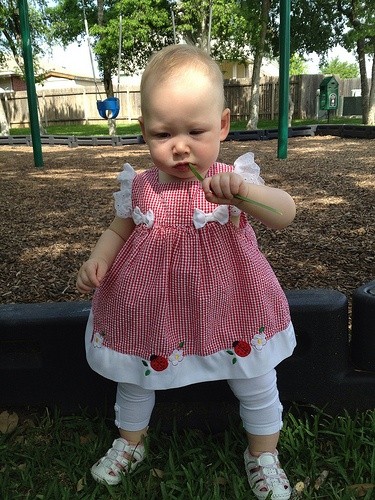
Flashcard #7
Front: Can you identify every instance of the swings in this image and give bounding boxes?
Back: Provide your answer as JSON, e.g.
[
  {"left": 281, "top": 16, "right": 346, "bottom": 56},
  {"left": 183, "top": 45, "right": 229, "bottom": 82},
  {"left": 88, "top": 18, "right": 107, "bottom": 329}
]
[
  {"left": 82, "top": 0, "right": 124, "bottom": 121},
  {"left": 169, "top": 0, "right": 214, "bottom": 59}
]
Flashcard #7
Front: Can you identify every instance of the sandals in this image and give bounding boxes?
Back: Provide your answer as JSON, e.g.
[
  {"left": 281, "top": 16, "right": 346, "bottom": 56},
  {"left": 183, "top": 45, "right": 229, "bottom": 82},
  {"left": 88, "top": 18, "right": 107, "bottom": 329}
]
[
  {"left": 91, "top": 438, "right": 146, "bottom": 486},
  {"left": 243, "top": 447, "right": 292, "bottom": 500}
]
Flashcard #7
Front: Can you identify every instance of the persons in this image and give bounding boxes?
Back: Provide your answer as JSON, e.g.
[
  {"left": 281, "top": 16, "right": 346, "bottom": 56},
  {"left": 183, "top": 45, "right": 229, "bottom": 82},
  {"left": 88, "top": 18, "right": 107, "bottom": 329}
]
[{"left": 76, "top": 45, "right": 295, "bottom": 499}]
